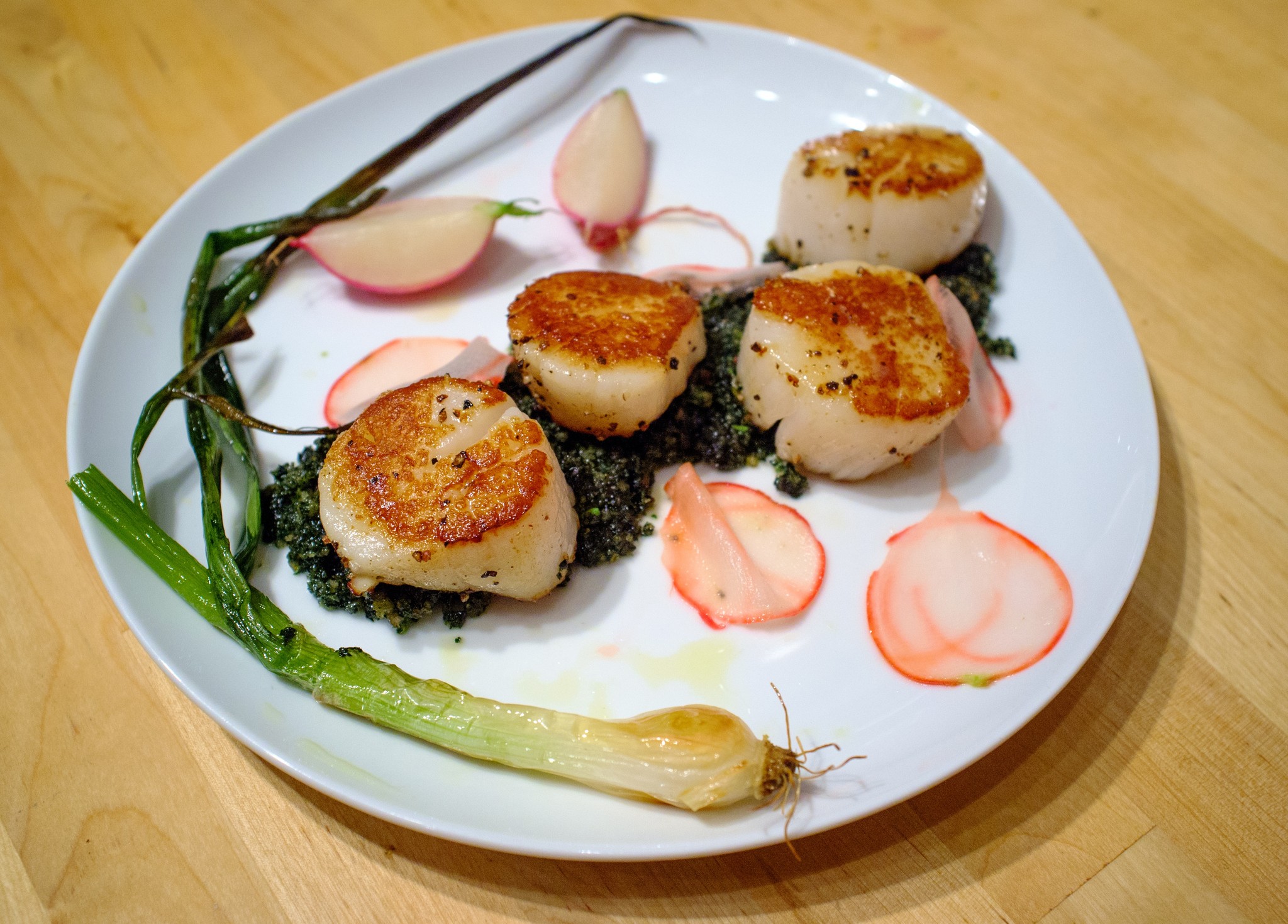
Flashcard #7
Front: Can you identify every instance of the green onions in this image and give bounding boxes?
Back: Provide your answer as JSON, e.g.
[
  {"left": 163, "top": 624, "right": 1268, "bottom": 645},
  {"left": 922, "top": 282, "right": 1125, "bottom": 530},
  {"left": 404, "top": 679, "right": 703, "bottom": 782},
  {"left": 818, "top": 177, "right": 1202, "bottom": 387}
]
[{"left": 69, "top": 13, "right": 848, "bottom": 811}]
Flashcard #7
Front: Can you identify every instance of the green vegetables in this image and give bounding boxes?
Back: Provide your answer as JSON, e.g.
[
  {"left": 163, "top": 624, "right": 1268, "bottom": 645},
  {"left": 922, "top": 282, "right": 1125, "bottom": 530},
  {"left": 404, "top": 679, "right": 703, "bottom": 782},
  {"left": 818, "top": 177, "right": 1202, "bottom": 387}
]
[{"left": 255, "top": 239, "right": 1016, "bottom": 630}]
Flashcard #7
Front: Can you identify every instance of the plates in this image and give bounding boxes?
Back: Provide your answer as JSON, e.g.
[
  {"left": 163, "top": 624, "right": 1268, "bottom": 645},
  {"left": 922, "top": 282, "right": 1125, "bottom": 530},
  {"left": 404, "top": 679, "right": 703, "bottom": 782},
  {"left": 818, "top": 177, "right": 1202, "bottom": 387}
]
[{"left": 67, "top": 13, "right": 1159, "bottom": 864}]
